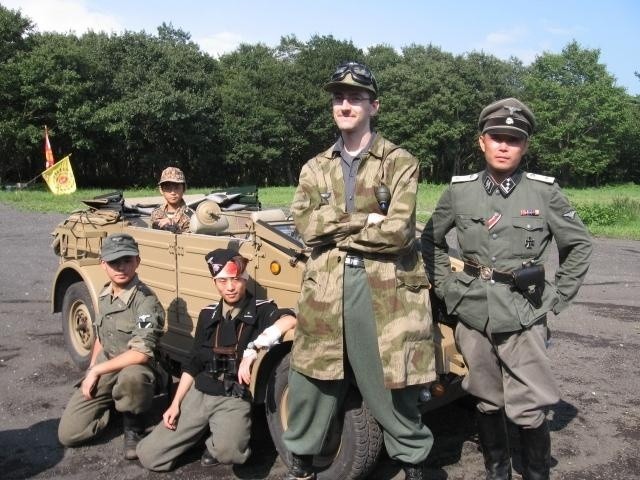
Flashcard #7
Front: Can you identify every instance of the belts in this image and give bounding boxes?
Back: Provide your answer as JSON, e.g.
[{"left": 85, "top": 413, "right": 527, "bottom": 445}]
[{"left": 464, "top": 263, "right": 514, "bottom": 285}]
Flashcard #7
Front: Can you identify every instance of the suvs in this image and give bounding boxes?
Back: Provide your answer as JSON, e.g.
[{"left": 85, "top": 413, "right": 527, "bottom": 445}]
[{"left": 52, "top": 188, "right": 469, "bottom": 476}]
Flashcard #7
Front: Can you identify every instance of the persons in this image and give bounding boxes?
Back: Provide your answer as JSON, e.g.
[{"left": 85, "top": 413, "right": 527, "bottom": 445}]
[
  {"left": 58, "top": 232, "right": 165, "bottom": 460},
  {"left": 135, "top": 249, "right": 296, "bottom": 473},
  {"left": 283, "top": 63, "right": 437, "bottom": 480},
  {"left": 149, "top": 167, "right": 196, "bottom": 232},
  {"left": 421, "top": 98, "right": 593, "bottom": 480}
]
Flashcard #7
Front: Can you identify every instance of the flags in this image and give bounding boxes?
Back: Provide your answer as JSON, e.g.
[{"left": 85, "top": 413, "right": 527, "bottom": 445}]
[
  {"left": 45, "top": 125, "right": 54, "bottom": 169},
  {"left": 42, "top": 156, "right": 77, "bottom": 196}
]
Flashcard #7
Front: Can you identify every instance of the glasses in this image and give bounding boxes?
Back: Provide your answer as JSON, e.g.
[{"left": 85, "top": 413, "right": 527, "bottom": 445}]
[
  {"left": 327, "top": 92, "right": 370, "bottom": 102},
  {"left": 329, "top": 62, "right": 373, "bottom": 85}
]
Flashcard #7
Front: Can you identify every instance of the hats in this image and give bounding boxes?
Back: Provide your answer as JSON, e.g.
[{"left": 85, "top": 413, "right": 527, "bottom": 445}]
[
  {"left": 158, "top": 166, "right": 184, "bottom": 184},
  {"left": 478, "top": 97, "right": 536, "bottom": 140},
  {"left": 323, "top": 62, "right": 375, "bottom": 92},
  {"left": 102, "top": 232, "right": 139, "bottom": 262}
]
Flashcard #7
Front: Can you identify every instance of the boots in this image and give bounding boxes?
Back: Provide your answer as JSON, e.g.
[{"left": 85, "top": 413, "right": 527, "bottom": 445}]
[
  {"left": 201, "top": 449, "right": 221, "bottom": 467},
  {"left": 476, "top": 409, "right": 513, "bottom": 480},
  {"left": 404, "top": 459, "right": 426, "bottom": 480},
  {"left": 285, "top": 453, "right": 317, "bottom": 480},
  {"left": 519, "top": 422, "right": 549, "bottom": 480},
  {"left": 120, "top": 411, "right": 142, "bottom": 461}
]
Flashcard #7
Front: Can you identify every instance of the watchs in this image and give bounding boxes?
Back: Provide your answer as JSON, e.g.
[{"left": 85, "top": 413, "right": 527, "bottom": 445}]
[{"left": 247, "top": 341, "right": 260, "bottom": 353}]
